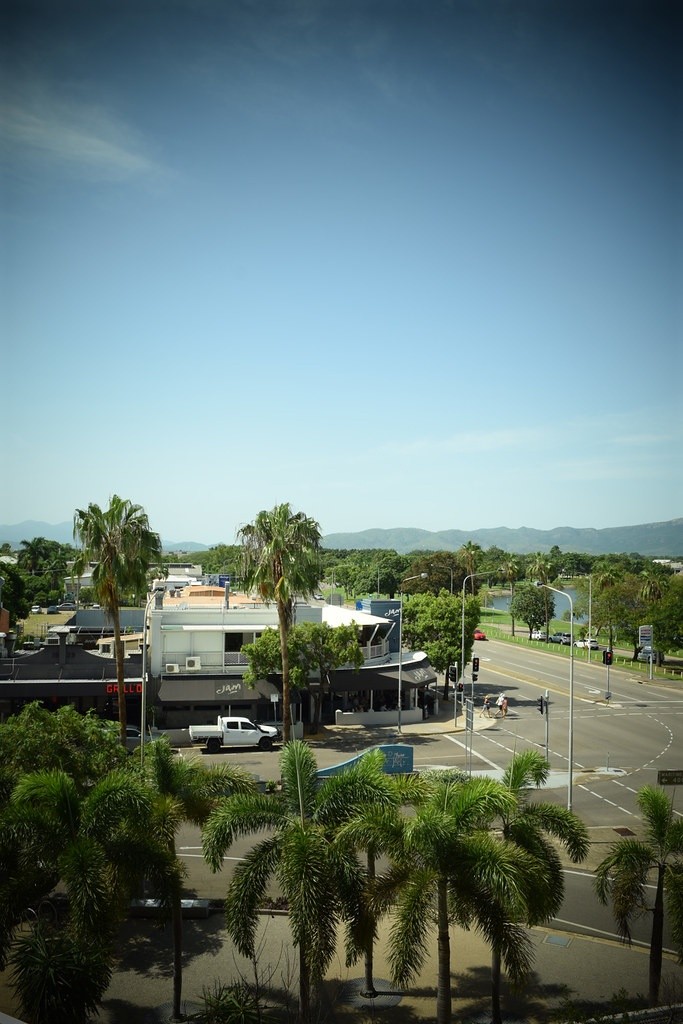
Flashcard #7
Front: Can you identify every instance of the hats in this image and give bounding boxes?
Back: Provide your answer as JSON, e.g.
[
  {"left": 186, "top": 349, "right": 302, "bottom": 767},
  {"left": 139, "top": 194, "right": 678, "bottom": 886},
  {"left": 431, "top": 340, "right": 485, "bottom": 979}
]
[{"left": 500, "top": 693, "right": 505, "bottom": 697}]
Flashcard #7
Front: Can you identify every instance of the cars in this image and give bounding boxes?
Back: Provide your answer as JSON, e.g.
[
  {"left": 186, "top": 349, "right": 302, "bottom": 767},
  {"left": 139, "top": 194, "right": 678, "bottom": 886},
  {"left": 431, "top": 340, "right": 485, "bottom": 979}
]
[
  {"left": 528, "top": 631, "right": 546, "bottom": 640},
  {"left": 638, "top": 649, "right": 665, "bottom": 662},
  {"left": 104, "top": 725, "right": 161, "bottom": 752},
  {"left": 474, "top": 630, "right": 486, "bottom": 640},
  {"left": 31, "top": 603, "right": 76, "bottom": 614},
  {"left": 316, "top": 594, "right": 324, "bottom": 600}
]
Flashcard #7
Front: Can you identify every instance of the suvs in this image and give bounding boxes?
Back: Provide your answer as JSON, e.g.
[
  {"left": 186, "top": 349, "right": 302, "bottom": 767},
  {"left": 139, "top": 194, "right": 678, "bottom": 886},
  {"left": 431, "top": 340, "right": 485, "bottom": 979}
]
[
  {"left": 574, "top": 639, "right": 599, "bottom": 650},
  {"left": 548, "top": 632, "right": 571, "bottom": 645}
]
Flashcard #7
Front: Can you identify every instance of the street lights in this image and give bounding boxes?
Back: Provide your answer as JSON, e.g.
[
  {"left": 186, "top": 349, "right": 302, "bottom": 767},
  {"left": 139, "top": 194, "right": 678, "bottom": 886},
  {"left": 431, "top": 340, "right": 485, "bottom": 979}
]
[
  {"left": 534, "top": 580, "right": 573, "bottom": 812},
  {"left": 430, "top": 564, "right": 453, "bottom": 595},
  {"left": 140, "top": 587, "right": 165, "bottom": 767},
  {"left": 462, "top": 568, "right": 507, "bottom": 706},
  {"left": 398, "top": 572, "right": 429, "bottom": 734}
]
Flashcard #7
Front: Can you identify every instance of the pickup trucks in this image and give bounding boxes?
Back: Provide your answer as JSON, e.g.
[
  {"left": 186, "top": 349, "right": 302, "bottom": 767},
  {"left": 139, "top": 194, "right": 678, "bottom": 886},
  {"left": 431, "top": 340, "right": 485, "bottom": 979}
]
[{"left": 189, "top": 716, "right": 278, "bottom": 752}]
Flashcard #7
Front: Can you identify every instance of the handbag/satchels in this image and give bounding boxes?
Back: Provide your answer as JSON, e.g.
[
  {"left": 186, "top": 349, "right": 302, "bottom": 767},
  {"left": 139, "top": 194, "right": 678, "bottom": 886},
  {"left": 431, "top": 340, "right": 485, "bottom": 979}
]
[{"left": 496, "top": 699, "right": 498, "bottom": 704}]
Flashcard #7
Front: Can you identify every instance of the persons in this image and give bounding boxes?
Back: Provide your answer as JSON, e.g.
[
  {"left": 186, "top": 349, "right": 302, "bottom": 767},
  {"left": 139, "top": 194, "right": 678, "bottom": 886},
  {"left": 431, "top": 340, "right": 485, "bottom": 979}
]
[
  {"left": 495, "top": 693, "right": 508, "bottom": 718},
  {"left": 352, "top": 695, "right": 393, "bottom": 712},
  {"left": 479, "top": 695, "right": 491, "bottom": 718}
]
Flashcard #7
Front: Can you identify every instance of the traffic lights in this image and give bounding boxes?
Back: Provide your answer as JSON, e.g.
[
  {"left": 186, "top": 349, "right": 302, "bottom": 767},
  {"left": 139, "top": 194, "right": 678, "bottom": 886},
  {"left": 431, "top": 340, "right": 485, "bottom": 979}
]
[
  {"left": 537, "top": 695, "right": 543, "bottom": 715},
  {"left": 473, "top": 658, "right": 479, "bottom": 672},
  {"left": 459, "top": 683, "right": 463, "bottom": 692},
  {"left": 450, "top": 667, "right": 456, "bottom": 682},
  {"left": 473, "top": 674, "right": 478, "bottom": 682},
  {"left": 605, "top": 652, "right": 612, "bottom": 665}
]
